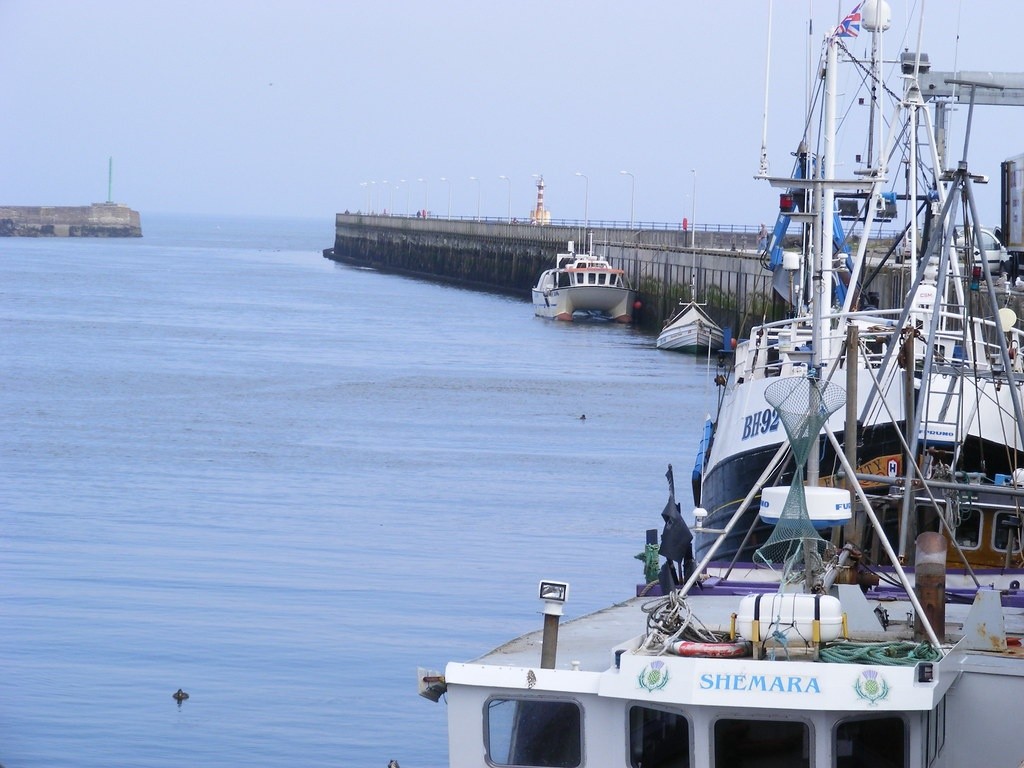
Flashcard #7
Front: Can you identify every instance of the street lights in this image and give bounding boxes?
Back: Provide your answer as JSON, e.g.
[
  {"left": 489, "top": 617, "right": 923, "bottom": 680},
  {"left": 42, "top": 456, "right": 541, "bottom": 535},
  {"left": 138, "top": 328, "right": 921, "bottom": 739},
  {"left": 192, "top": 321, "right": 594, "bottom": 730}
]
[
  {"left": 499, "top": 176, "right": 510, "bottom": 224},
  {"left": 401, "top": 180, "right": 409, "bottom": 218},
  {"left": 532, "top": 175, "right": 544, "bottom": 226},
  {"left": 619, "top": 171, "right": 634, "bottom": 233},
  {"left": 418, "top": 179, "right": 427, "bottom": 213},
  {"left": 470, "top": 177, "right": 480, "bottom": 223},
  {"left": 691, "top": 168, "right": 696, "bottom": 248},
  {"left": 360, "top": 181, "right": 393, "bottom": 216},
  {"left": 577, "top": 173, "right": 589, "bottom": 225},
  {"left": 441, "top": 178, "right": 451, "bottom": 220}
]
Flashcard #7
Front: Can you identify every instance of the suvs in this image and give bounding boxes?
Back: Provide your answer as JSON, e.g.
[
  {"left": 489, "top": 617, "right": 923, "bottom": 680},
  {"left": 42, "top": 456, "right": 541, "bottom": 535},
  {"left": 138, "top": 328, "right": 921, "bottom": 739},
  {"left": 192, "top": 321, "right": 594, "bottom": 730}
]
[{"left": 895, "top": 227, "right": 921, "bottom": 262}]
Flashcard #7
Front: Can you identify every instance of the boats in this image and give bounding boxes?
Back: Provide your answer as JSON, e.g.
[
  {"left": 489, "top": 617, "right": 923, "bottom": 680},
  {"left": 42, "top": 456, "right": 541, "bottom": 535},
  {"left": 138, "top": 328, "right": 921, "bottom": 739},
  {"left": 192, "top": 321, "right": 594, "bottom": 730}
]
[
  {"left": 655, "top": 293, "right": 724, "bottom": 356},
  {"left": 411, "top": 0, "right": 1024, "bottom": 768},
  {"left": 530, "top": 234, "right": 632, "bottom": 326},
  {"left": 693, "top": 0, "right": 1023, "bottom": 571}
]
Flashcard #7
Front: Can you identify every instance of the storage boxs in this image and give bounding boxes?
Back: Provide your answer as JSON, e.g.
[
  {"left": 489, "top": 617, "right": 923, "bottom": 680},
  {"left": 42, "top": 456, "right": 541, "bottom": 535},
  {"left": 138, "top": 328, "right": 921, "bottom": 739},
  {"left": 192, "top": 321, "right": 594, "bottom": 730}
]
[{"left": 736, "top": 592, "right": 844, "bottom": 641}]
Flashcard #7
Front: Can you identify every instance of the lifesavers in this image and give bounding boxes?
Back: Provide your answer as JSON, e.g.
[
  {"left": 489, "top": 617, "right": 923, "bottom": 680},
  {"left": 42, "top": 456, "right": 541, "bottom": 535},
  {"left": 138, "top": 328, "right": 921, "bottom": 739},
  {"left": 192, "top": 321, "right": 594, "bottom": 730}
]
[{"left": 665, "top": 638, "right": 753, "bottom": 659}]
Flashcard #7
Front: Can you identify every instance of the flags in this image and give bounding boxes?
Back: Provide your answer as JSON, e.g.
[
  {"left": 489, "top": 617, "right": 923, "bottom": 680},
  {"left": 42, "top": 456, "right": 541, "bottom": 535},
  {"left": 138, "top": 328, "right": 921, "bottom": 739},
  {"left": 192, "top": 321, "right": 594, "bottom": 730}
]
[{"left": 830, "top": 2, "right": 864, "bottom": 37}]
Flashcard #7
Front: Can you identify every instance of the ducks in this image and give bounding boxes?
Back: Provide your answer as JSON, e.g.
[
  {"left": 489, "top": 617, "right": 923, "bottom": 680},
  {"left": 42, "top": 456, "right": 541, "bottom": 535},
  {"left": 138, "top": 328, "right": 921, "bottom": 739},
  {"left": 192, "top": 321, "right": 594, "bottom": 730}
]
[
  {"left": 579, "top": 414, "right": 586, "bottom": 419},
  {"left": 172, "top": 689, "right": 189, "bottom": 699}
]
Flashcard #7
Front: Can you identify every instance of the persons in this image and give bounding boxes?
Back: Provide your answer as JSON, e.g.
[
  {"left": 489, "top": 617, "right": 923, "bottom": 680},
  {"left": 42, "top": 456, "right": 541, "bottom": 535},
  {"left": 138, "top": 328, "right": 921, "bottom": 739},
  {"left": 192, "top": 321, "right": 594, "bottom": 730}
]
[
  {"left": 757, "top": 223, "right": 767, "bottom": 254},
  {"left": 416, "top": 209, "right": 421, "bottom": 218},
  {"left": 422, "top": 209, "right": 427, "bottom": 219},
  {"left": 345, "top": 209, "right": 349, "bottom": 214}
]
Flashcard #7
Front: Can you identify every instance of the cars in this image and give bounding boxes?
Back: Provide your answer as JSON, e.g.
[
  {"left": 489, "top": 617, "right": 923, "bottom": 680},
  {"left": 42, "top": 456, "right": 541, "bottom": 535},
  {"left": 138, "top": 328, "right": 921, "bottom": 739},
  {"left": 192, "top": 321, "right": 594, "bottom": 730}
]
[{"left": 955, "top": 225, "right": 1007, "bottom": 276}]
